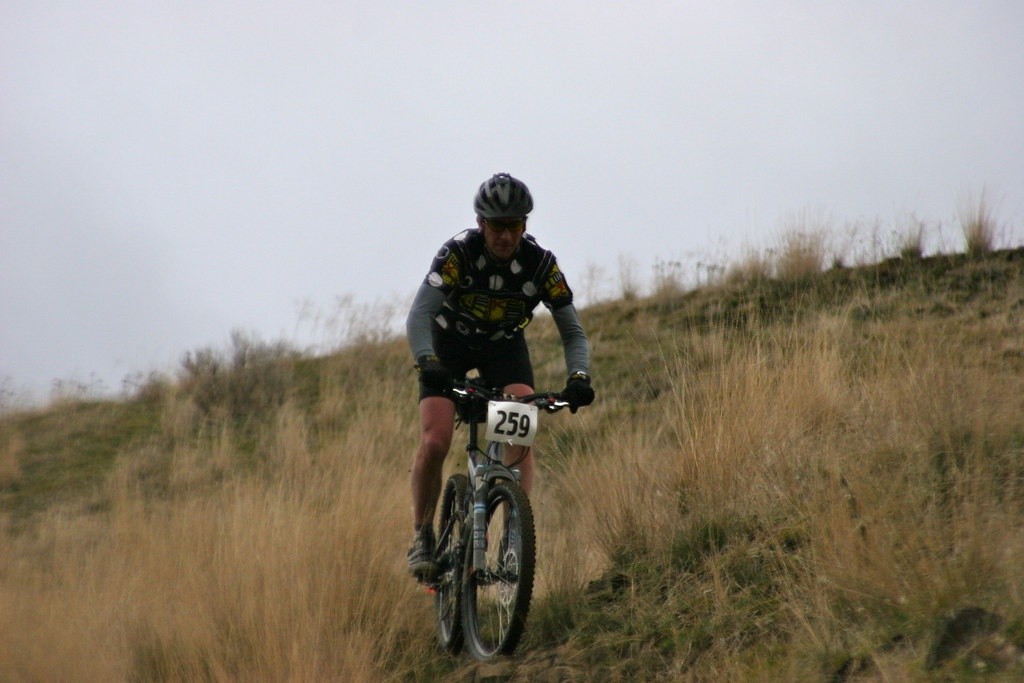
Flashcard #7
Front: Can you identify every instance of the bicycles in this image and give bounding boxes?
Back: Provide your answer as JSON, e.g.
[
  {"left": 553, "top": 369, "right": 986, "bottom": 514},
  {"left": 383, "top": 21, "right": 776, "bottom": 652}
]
[{"left": 411, "top": 362, "right": 597, "bottom": 663}]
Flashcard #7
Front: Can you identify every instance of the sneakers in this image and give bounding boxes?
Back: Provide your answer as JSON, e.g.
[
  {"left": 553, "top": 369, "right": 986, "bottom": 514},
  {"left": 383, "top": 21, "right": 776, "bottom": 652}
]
[
  {"left": 489, "top": 555, "right": 517, "bottom": 583},
  {"left": 408, "top": 533, "right": 439, "bottom": 578}
]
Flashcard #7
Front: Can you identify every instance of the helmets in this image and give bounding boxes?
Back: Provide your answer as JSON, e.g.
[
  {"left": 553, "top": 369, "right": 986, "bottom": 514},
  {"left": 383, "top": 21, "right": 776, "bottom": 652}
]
[{"left": 473, "top": 173, "right": 533, "bottom": 220}]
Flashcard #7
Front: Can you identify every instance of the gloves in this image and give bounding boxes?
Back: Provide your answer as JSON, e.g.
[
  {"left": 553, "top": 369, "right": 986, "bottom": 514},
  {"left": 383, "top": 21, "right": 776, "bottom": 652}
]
[
  {"left": 418, "top": 362, "right": 449, "bottom": 390},
  {"left": 558, "top": 378, "right": 594, "bottom": 414}
]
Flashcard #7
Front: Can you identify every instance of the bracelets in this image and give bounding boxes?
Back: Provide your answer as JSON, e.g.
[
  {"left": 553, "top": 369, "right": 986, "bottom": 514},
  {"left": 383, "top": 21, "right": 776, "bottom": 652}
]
[{"left": 573, "top": 371, "right": 592, "bottom": 383}]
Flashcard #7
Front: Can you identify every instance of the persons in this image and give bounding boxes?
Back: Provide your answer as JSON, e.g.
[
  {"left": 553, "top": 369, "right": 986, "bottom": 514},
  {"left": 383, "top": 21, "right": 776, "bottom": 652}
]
[{"left": 406, "top": 173, "right": 594, "bottom": 579}]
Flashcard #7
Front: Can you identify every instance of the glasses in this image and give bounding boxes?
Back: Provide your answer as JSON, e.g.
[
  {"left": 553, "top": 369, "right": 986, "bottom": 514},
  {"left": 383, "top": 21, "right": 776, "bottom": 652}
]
[{"left": 482, "top": 216, "right": 527, "bottom": 232}]
